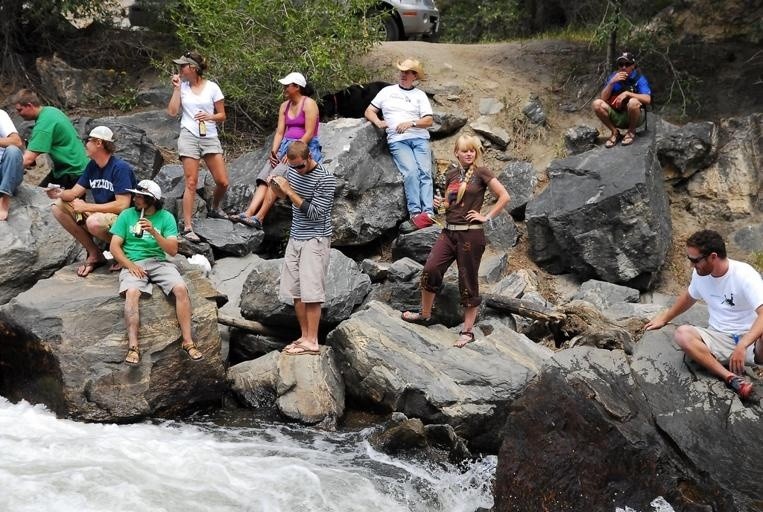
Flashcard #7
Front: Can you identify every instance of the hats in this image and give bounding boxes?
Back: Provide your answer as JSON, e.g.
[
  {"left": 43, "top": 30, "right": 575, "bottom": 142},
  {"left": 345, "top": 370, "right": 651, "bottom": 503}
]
[
  {"left": 616, "top": 52, "right": 634, "bottom": 64},
  {"left": 171, "top": 55, "right": 199, "bottom": 69},
  {"left": 77, "top": 126, "right": 117, "bottom": 143},
  {"left": 394, "top": 57, "right": 425, "bottom": 80},
  {"left": 277, "top": 72, "right": 306, "bottom": 89},
  {"left": 125, "top": 180, "right": 162, "bottom": 201}
]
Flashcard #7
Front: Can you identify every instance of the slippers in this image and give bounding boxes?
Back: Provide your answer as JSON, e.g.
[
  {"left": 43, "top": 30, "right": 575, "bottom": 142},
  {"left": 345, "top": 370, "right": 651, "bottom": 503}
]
[{"left": 282, "top": 341, "right": 321, "bottom": 355}]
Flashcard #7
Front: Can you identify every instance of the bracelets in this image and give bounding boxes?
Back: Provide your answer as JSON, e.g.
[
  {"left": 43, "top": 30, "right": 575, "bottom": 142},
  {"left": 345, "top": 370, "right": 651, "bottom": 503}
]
[
  {"left": 485, "top": 215, "right": 491, "bottom": 222},
  {"left": 412, "top": 121, "right": 416, "bottom": 127}
]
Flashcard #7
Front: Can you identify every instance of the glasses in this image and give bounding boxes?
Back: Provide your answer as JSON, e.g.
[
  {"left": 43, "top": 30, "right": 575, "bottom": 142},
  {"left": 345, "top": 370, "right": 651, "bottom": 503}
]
[
  {"left": 686, "top": 255, "right": 704, "bottom": 264},
  {"left": 286, "top": 160, "right": 308, "bottom": 169},
  {"left": 132, "top": 183, "right": 156, "bottom": 199},
  {"left": 619, "top": 61, "right": 631, "bottom": 67}
]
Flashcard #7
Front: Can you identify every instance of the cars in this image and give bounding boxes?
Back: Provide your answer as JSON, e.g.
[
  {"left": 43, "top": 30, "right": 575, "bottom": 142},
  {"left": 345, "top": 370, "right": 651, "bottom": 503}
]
[{"left": 365, "top": 0, "right": 442, "bottom": 41}]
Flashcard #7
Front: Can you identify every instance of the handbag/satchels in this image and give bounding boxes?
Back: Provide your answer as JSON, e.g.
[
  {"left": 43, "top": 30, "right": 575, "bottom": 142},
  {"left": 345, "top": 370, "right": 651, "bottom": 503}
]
[
  {"left": 604, "top": 84, "right": 639, "bottom": 112},
  {"left": 399, "top": 211, "right": 432, "bottom": 233}
]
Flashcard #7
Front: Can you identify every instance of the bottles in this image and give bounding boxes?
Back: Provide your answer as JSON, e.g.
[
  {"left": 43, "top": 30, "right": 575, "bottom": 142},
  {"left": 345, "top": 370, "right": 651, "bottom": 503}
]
[
  {"left": 270, "top": 150, "right": 280, "bottom": 165},
  {"left": 197, "top": 110, "right": 207, "bottom": 137},
  {"left": 131, "top": 208, "right": 144, "bottom": 239},
  {"left": 24, "top": 137, "right": 36, "bottom": 166},
  {"left": 71, "top": 193, "right": 85, "bottom": 224},
  {"left": 435, "top": 188, "right": 446, "bottom": 223}
]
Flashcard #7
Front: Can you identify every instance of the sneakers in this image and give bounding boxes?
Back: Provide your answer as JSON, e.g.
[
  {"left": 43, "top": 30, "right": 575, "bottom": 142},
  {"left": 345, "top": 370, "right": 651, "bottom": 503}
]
[{"left": 727, "top": 376, "right": 753, "bottom": 396}]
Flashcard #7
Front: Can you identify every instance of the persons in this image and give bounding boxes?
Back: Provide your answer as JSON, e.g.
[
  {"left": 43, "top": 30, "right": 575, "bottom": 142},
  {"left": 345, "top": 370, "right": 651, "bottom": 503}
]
[
  {"left": 642, "top": 229, "right": 763, "bottom": 400},
  {"left": 10, "top": 88, "right": 90, "bottom": 200},
  {"left": 107, "top": 179, "right": 205, "bottom": 367},
  {"left": 363, "top": 59, "right": 434, "bottom": 229},
  {"left": 266, "top": 140, "right": 337, "bottom": 357},
  {"left": 591, "top": 51, "right": 651, "bottom": 148},
  {"left": 401, "top": 131, "right": 510, "bottom": 348},
  {"left": 42, "top": 126, "right": 137, "bottom": 278},
  {"left": 229, "top": 72, "right": 320, "bottom": 230},
  {"left": 0, "top": 108, "right": 25, "bottom": 221},
  {"left": 166, "top": 51, "right": 230, "bottom": 244}
]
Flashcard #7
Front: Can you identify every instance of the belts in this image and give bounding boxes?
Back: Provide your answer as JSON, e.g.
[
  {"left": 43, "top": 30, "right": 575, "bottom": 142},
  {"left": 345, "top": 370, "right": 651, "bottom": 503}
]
[{"left": 445, "top": 223, "right": 484, "bottom": 231}]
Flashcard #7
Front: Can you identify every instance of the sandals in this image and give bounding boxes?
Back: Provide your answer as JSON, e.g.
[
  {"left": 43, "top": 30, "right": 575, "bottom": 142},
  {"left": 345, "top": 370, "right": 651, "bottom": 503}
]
[
  {"left": 180, "top": 340, "right": 204, "bottom": 363},
  {"left": 211, "top": 205, "right": 229, "bottom": 219},
  {"left": 77, "top": 260, "right": 100, "bottom": 276},
  {"left": 401, "top": 310, "right": 432, "bottom": 326},
  {"left": 230, "top": 213, "right": 262, "bottom": 229},
  {"left": 605, "top": 129, "right": 620, "bottom": 147},
  {"left": 181, "top": 230, "right": 200, "bottom": 241},
  {"left": 453, "top": 331, "right": 475, "bottom": 348},
  {"left": 621, "top": 129, "right": 636, "bottom": 146},
  {"left": 125, "top": 344, "right": 142, "bottom": 366},
  {"left": 109, "top": 260, "right": 122, "bottom": 271}
]
